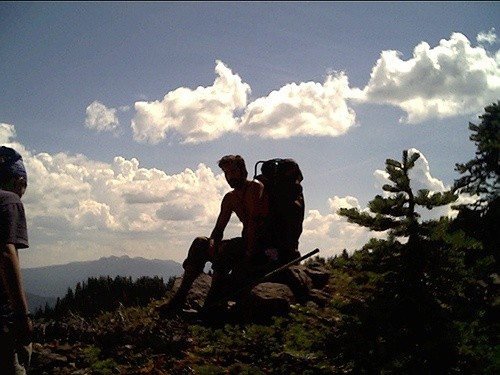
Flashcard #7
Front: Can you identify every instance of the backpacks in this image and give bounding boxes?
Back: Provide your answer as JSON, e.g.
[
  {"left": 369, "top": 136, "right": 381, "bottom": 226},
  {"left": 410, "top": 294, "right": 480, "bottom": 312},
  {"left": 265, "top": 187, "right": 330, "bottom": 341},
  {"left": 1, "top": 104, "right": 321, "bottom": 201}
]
[{"left": 252, "top": 153, "right": 307, "bottom": 250}]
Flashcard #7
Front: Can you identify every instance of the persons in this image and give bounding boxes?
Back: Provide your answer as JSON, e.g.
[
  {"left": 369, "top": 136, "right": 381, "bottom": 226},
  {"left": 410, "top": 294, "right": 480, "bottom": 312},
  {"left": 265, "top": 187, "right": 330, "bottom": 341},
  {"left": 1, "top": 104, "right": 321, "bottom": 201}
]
[
  {"left": 0, "top": 145, "right": 35, "bottom": 373},
  {"left": 208, "top": 158, "right": 304, "bottom": 320},
  {"left": 157, "top": 156, "right": 263, "bottom": 316}
]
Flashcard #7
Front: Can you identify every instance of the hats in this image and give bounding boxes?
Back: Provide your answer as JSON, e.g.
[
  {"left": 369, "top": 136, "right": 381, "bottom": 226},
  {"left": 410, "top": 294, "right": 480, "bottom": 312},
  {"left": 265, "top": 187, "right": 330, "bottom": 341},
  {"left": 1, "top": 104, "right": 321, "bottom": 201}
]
[{"left": 0, "top": 145, "right": 28, "bottom": 179}]
